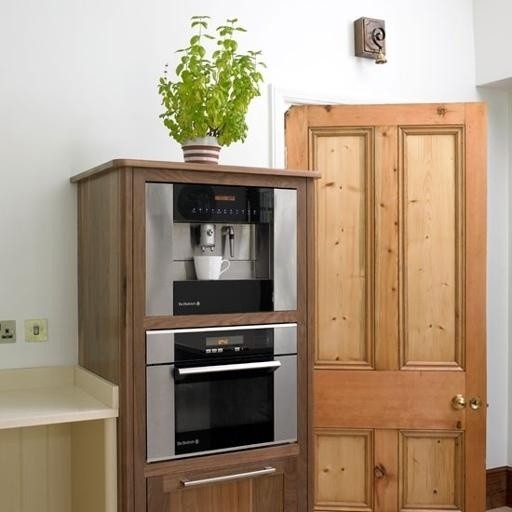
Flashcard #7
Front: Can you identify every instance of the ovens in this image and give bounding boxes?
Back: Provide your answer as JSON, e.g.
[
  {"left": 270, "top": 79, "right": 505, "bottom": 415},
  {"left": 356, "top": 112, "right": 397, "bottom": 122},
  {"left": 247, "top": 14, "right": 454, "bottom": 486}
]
[{"left": 147, "top": 323, "right": 297, "bottom": 463}]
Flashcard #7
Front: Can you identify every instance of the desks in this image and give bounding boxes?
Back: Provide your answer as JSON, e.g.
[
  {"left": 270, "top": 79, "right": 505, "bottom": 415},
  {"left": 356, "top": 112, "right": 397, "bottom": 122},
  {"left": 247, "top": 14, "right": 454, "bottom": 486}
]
[{"left": 0, "top": 364, "right": 119, "bottom": 512}]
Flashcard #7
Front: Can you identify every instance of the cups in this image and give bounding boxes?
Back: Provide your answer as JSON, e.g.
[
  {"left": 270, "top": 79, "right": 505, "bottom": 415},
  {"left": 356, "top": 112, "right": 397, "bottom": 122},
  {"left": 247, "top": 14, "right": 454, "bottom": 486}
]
[{"left": 193, "top": 255, "right": 230, "bottom": 281}]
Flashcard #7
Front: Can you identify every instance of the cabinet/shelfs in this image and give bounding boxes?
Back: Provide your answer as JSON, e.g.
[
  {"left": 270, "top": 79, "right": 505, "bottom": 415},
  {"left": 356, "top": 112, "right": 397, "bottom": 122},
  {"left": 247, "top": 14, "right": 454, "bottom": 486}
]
[{"left": 70, "top": 158, "right": 321, "bottom": 511}]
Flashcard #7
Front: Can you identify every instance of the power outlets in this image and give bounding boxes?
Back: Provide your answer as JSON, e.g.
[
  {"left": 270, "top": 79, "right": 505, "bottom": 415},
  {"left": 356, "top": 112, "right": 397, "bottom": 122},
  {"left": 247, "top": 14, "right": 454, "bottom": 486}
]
[{"left": 0, "top": 320, "right": 17, "bottom": 344}]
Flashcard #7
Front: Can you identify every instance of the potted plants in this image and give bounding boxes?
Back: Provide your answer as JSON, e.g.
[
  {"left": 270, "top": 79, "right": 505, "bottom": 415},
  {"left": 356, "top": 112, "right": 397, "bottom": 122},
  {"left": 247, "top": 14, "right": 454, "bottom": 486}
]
[{"left": 156, "top": 15, "right": 268, "bottom": 164}]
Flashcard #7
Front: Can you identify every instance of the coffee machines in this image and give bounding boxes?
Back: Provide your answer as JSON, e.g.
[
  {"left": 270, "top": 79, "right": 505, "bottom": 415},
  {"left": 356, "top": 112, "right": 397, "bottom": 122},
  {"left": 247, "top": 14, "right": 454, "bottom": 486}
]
[{"left": 146, "top": 183, "right": 297, "bottom": 316}]
